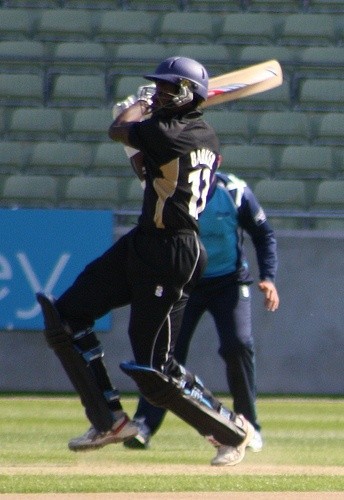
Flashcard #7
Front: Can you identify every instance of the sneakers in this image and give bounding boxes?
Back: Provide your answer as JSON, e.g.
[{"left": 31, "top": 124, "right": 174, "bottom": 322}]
[
  {"left": 68, "top": 413, "right": 140, "bottom": 453},
  {"left": 210, "top": 415, "right": 256, "bottom": 468}
]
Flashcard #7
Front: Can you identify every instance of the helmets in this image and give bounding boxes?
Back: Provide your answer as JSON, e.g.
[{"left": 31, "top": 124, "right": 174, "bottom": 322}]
[{"left": 142, "top": 56, "right": 208, "bottom": 106}]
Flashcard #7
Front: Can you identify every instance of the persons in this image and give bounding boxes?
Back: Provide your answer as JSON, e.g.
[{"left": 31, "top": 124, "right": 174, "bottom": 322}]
[
  {"left": 36, "top": 56, "right": 267, "bottom": 465},
  {"left": 123, "top": 152, "right": 280, "bottom": 450}
]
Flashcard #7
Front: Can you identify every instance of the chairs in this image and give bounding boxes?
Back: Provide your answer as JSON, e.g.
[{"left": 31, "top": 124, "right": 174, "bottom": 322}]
[{"left": 0, "top": 0, "right": 344, "bottom": 232}]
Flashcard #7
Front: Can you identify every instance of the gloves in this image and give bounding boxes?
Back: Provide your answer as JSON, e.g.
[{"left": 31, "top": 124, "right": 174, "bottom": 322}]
[
  {"left": 112, "top": 94, "right": 147, "bottom": 158},
  {"left": 136, "top": 82, "right": 158, "bottom": 106}
]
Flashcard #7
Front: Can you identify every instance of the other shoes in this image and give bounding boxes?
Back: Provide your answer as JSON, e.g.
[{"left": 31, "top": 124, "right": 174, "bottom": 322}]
[{"left": 123, "top": 419, "right": 152, "bottom": 451}]
